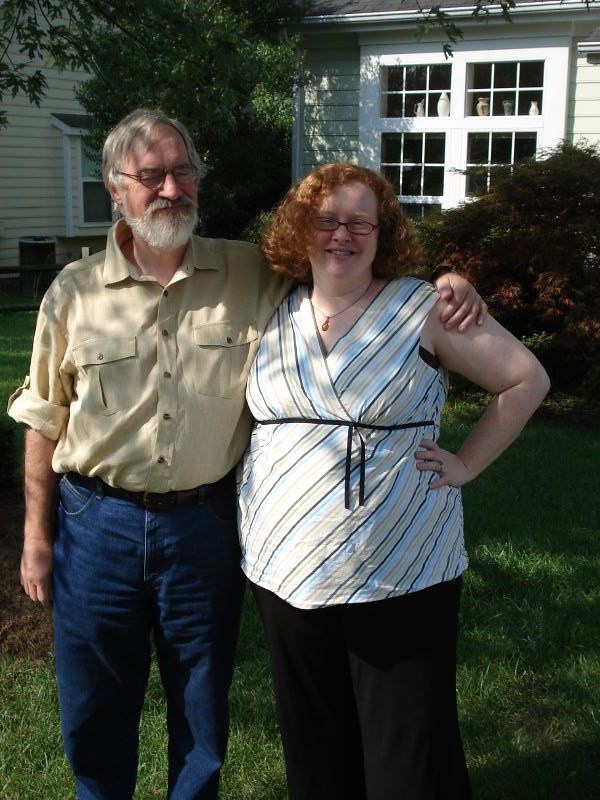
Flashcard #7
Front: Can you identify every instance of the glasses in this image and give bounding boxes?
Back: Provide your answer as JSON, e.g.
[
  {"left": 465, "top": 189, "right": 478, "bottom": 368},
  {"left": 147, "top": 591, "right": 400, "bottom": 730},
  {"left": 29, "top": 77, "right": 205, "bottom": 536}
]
[
  {"left": 309, "top": 216, "right": 380, "bottom": 236},
  {"left": 115, "top": 162, "right": 198, "bottom": 189}
]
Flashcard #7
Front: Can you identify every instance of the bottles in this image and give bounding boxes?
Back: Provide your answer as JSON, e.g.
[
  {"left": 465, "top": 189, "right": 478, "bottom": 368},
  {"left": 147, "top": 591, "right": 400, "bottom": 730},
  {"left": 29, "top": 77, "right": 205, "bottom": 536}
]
[
  {"left": 437, "top": 93, "right": 449, "bottom": 117},
  {"left": 477, "top": 97, "right": 489, "bottom": 116},
  {"left": 529, "top": 101, "right": 539, "bottom": 116},
  {"left": 503, "top": 100, "right": 513, "bottom": 116}
]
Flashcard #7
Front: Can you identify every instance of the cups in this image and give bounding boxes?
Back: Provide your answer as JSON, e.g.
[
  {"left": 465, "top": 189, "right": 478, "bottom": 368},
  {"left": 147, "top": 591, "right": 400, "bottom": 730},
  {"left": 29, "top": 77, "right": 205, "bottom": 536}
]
[{"left": 414, "top": 103, "right": 425, "bottom": 117}]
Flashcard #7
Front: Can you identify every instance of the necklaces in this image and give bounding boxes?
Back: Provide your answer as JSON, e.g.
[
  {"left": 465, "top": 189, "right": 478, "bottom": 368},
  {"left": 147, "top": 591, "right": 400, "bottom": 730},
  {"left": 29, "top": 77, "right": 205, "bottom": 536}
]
[{"left": 309, "top": 278, "right": 373, "bottom": 331}]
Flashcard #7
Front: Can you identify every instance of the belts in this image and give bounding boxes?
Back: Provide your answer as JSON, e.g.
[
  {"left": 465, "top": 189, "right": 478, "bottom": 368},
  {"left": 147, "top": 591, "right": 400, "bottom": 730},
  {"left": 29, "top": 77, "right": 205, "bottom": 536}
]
[{"left": 67, "top": 472, "right": 235, "bottom": 513}]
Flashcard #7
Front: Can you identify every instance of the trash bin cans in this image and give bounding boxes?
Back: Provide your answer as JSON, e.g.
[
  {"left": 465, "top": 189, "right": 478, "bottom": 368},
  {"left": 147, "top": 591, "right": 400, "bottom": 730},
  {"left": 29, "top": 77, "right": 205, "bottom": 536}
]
[{"left": 18, "top": 240, "right": 57, "bottom": 297}]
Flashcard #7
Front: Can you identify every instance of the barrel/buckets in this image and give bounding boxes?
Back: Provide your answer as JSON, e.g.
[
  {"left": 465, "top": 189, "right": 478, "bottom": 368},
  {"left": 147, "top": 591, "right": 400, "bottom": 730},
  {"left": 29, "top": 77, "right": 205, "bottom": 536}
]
[{"left": 19, "top": 236, "right": 56, "bottom": 297}]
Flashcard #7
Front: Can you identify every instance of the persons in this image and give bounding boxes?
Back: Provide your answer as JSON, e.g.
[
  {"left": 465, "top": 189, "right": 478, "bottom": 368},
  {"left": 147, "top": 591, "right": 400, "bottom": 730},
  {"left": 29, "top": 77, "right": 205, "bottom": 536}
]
[
  {"left": 235, "top": 163, "right": 551, "bottom": 800},
  {"left": 7, "top": 108, "right": 487, "bottom": 800}
]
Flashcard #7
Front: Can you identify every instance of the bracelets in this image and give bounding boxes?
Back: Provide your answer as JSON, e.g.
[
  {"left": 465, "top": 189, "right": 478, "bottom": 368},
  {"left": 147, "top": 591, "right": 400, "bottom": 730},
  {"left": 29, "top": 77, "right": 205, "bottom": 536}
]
[{"left": 428, "top": 265, "right": 455, "bottom": 283}]
